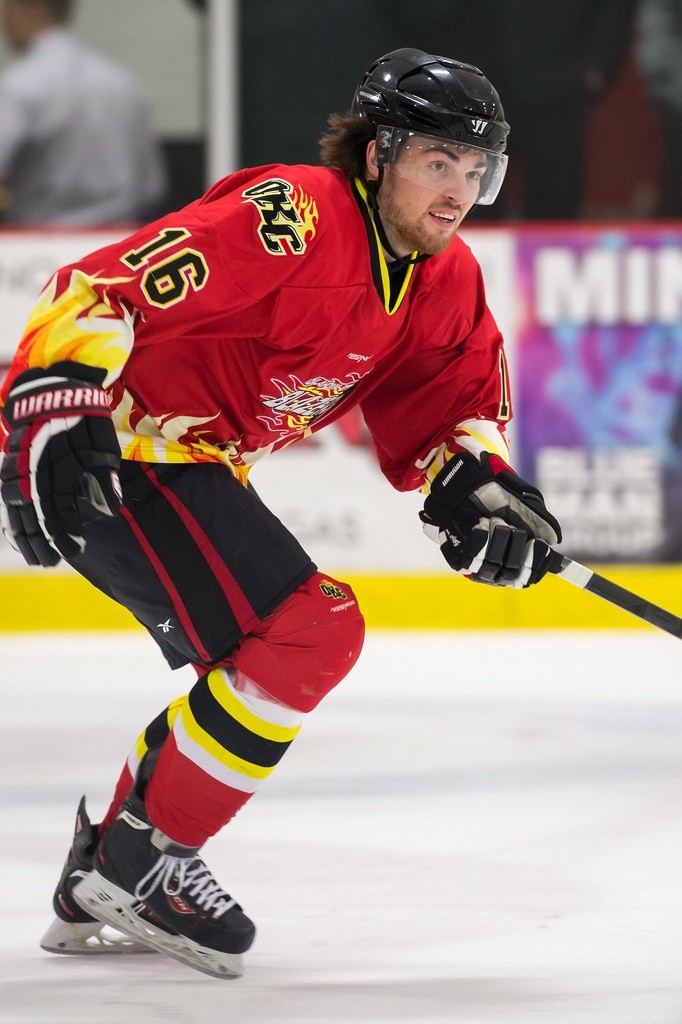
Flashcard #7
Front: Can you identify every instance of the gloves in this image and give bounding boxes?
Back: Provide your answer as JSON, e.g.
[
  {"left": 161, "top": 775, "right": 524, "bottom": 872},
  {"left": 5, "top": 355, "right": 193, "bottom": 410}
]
[
  {"left": 419, "top": 451, "right": 562, "bottom": 588},
  {"left": 0, "top": 360, "right": 126, "bottom": 568}
]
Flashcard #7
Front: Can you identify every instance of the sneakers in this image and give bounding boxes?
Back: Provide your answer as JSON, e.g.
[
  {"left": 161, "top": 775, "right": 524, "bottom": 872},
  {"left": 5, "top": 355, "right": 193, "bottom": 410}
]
[
  {"left": 40, "top": 794, "right": 160, "bottom": 954},
  {"left": 72, "top": 743, "right": 255, "bottom": 979}
]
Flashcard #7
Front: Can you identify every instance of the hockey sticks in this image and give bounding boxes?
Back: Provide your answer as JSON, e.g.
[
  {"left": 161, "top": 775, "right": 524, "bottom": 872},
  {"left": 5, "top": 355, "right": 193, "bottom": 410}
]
[{"left": 547, "top": 544, "right": 681, "bottom": 640}]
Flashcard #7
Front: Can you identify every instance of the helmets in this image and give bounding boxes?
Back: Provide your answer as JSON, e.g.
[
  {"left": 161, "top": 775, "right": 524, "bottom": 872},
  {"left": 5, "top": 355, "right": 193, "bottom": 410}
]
[{"left": 350, "top": 47, "right": 510, "bottom": 153}]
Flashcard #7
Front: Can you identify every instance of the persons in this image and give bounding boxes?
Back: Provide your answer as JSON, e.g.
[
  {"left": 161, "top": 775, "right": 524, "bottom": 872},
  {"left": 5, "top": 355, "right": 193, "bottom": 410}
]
[
  {"left": 1, "top": 45, "right": 561, "bottom": 977},
  {"left": 577, "top": 38, "right": 662, "bottom": 219},
  {"left": 0, "top": 0, "right": 168, "bottom": 229}
]
